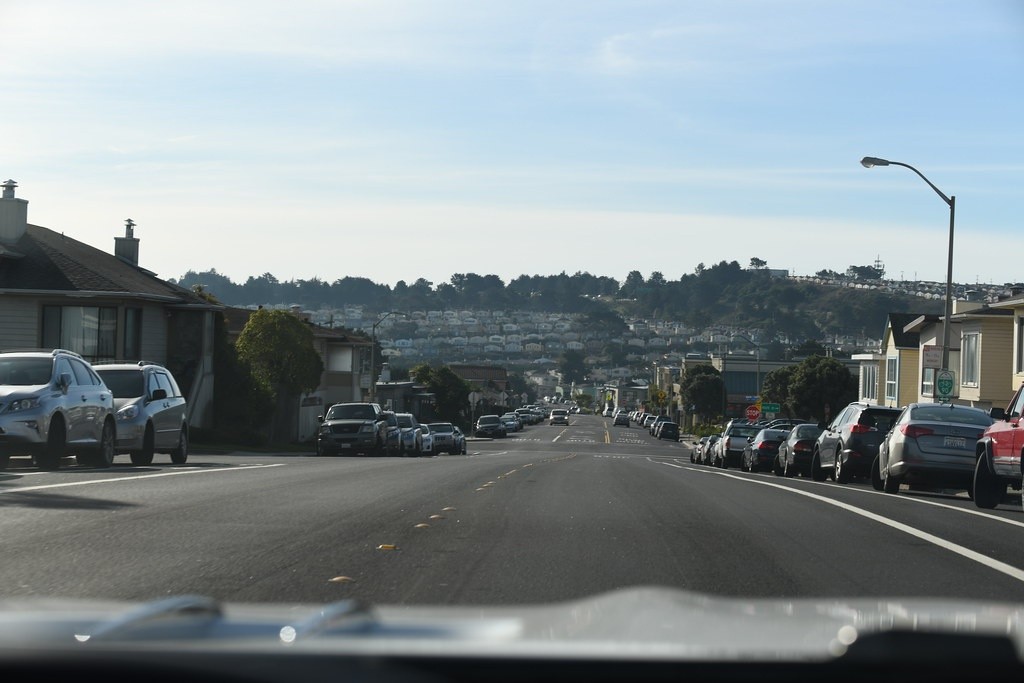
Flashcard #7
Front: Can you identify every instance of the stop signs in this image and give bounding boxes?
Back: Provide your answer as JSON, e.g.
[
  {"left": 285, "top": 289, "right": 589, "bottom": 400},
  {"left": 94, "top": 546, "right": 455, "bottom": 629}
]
[{"left": 745, "top": 406, "right": 759, "bottom": 420}]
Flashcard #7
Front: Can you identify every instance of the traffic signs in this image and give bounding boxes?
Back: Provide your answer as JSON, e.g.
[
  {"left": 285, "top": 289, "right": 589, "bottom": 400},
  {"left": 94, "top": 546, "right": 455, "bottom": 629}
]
[
  {"left": 938, "top": 396, "right": 950, "bottom": 401},
  {"left": 761, "top": 403, "right": 780, "bottom": 413}
]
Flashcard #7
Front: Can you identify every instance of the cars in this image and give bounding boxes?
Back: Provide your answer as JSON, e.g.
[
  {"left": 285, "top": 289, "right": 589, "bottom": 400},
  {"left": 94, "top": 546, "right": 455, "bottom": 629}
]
[
  {"left": 740, "top": 428, "right": 790, "bottom": 473},
  {"left": 545, "top": 395, "right": 570, "bottom": 405},
  {"left": 628, "top": 411, "right": 643, "bottom": 422},
  {"left": 973, "top": 383, "right": 1024, "bottom": 513},
  {"left": 617, "top": 410, "right": 627, "bottom": 416},
  {"left": 871, "top": 402, "right": 996, "bottom": 498},
  {"left": 774, "top": 423, "right": 825, "bottom": 478},
  {"left": 570, "top": 407, "right": 582, "bottom": 414},
  {"left": 476, "top": 415, "right": 506, "bottom": 438},
  {"left": 420, "top": 424, "right": 436, "bottom": 456},
  {"left": 690, "top": 434, "right": 720, "bottom": 465},
  {"left": 727, "top": 417, "right": 808, "bottom": 430},
  {"left": 614, "top": 414, "right": 630, "bottom": 428},
  {"left": 454, "top": 426, "right": 467, "bottom": 455},
  {"left": 654, "top": 421, "right": 662, "bottom": 437},
  {"left": 530, "top": 407, "right": 548, "bottom": 423}
]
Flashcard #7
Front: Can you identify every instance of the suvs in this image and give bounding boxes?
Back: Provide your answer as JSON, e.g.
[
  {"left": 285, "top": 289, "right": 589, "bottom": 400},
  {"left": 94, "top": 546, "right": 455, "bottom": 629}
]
[
  {"left": 317, "top": 402, "right": 390, "bottom": 457},
  {"left": 550, "top": 408, "right": 568, "bottom": 425},
  {"left": 811, "top": 401, "right": 908, "bottom": 484},
  {"left": 639, "top": 413, "right": 650, "bottom": 425},
  {"left": 0, "top": 348, "right": 117, "bottom": 471},
  {"left": 88, "top": 359, "right": 190, "bottom": 466},
  {"left": 657, "top": 422, "right": 679, "bottom": 441},
  {"left": 715, "top": 423, "right": 764, "bottom": 469},
  {"left": 500, "top": 413, "right": 522, "bottom": 432},
  {"left": 383, "top": 411, "right": 404, "bottom": 457},
  {"left": 395, "top": 413, "right": 423, "bottom": 457},
  {"left": 427, "top": 422, "right": 460, "bottom": 456},
  {"left": 644, "top": 416, "right": 656, "bottom": 428}
]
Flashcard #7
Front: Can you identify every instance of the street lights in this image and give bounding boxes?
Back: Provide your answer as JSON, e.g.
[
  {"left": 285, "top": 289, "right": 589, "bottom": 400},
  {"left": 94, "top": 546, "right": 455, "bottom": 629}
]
[
  {"left": 730, "top": 332, "right": 760, "bottom": 395},
  {"left": 370, "top": 311, "right": 407, "bottom": 403},
  {"left": 861, "top": 156, "right": 955, "bottom": 403}
]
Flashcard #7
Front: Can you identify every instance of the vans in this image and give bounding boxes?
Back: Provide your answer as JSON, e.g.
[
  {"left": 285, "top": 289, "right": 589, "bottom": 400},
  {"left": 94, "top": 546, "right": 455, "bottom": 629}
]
[
  {"left": 650, "top": 415, "right": 672, "bottom": 436},
  {"left": 612, "top": 407, "right": 625, "bottom": 417},
  {"left": 515, "top": 408, "right": 532, "bottom": 425},
  {"left": 604, "top": 407, "right": 613, "bottom": 417}
]
